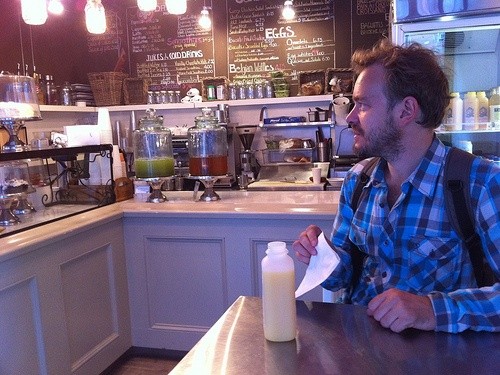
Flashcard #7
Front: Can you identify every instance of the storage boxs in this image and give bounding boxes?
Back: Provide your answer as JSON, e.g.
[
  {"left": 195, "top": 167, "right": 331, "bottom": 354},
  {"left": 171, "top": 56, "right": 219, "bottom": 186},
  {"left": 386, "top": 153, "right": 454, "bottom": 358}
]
[{"left": 63, "top": 124, "right": 100, "bottom": 147}]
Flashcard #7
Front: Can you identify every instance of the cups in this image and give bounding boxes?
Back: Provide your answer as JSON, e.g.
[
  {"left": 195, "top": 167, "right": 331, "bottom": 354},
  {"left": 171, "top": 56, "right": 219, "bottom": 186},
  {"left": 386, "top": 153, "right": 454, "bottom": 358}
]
[
  {"left": 332, "top": 96, "right": 351, "bottom": 126},
  {"left": 312, "top": 167, "right": 321, "bottom": 184},
  {"left": 318, "top": 140, "right": 329, "bottom": 162},
  {"left": 162, "top": 177, "right": 185, "bottom": 190}
]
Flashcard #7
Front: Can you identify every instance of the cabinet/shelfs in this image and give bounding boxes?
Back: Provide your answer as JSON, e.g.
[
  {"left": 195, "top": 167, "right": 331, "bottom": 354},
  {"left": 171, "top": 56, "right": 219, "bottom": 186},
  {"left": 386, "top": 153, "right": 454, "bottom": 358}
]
[
  {"left": 0, "top": 216, "right": 346, "bottom": 375},
  {"left": 247, "top": 121, "right": 336, "bottom": 192}
]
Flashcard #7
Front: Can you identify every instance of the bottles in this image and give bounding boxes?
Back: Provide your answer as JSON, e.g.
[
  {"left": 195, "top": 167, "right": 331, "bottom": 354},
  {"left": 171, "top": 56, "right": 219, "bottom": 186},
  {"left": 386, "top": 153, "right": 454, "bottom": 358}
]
[
  {"left": 132, "top": 108, "right": 176, "bottom": 180},
  {"left": 441, "top": 84, "right": 500, "bottom": 129},
  {"left": 28, "top": 73, "right": 72, "bottom": 106},
  {"left": 146, "top": 90, "right": 181, "bottom": 104},
  {"left": 188, "top": 108, "right": 228, "bottom": 176},
  {"left": 229, "top": 80, "right": 274, "bottom": 101},
  {"left": 261, "top": 239, "right": 298, "bottom": 342}
]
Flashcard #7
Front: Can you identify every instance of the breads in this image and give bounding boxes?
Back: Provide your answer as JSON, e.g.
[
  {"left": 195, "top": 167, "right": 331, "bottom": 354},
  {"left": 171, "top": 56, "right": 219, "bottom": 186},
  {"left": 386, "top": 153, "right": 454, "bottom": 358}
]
[
  {"left": 289, "top": 138, "right": 302, "bottom": 149},
  {"left": 301, "top": 82, "right": 322, "bottom": 95},
  {"left": 292, "top": 156, "right": 310, "bottom": 163},
  {"left": 331, "top": 78, "right": 353, "bottom": 93}
]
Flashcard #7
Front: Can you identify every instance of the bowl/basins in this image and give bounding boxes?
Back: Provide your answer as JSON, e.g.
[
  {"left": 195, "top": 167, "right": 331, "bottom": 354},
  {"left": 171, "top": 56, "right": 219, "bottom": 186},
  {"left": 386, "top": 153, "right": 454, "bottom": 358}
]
[{"left": 326, "top": 178, "right": 344, "bottom": 188}]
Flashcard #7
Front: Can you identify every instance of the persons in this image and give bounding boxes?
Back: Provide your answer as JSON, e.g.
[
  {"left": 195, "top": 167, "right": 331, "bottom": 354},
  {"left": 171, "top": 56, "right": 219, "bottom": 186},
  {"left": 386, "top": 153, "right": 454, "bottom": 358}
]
[{"left": 291, "top": 32, "right": 500, "bottom": 334}]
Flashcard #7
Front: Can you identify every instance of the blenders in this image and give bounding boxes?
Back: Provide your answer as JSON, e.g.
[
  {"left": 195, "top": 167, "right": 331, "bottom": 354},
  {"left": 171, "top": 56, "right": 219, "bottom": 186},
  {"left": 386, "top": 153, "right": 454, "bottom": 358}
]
[{"left": 235, "top": 123, "right": 260, "bottom": 183}]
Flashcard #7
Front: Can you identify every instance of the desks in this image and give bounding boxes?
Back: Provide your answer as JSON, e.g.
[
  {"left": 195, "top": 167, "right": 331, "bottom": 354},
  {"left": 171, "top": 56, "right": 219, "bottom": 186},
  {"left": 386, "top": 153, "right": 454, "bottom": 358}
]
[{"left": 168, "top": 296, "right": 500, "bottom": 375}]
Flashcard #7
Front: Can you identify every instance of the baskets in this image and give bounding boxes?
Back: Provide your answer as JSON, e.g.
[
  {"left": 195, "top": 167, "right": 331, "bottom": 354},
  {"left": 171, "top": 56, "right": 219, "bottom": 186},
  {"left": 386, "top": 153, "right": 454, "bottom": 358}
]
[
  {"left": 87, "top": 72, "right": 129, "bottom": 107},
  {"left": 297, "top": 69, "right": 325, "bottom": 96},
  {"left": 201, "top": 76, "right": 230, "bottom": 101},
  {"left": 123, "top": 77, "right": 149, "bottom": 105},
  {"left": 324, "top": 68, "right": 357, "bottom": 97}
]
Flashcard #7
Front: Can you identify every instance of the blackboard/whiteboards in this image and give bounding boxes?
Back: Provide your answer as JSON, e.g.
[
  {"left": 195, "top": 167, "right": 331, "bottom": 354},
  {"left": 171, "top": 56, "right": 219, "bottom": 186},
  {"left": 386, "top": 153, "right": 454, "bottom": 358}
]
[{"left": 45, "top": 0, "right": 397, "bottom": 101}]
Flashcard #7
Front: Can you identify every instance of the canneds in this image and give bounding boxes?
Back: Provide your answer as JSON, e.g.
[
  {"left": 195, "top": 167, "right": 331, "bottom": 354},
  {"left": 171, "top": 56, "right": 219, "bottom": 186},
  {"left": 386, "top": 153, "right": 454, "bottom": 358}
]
[
  {"left": 206, "top": 85, "right": 215, "bottom": 100},
  {"left": 217, "top": 84, "right": 225, "bottom": 99}
]
